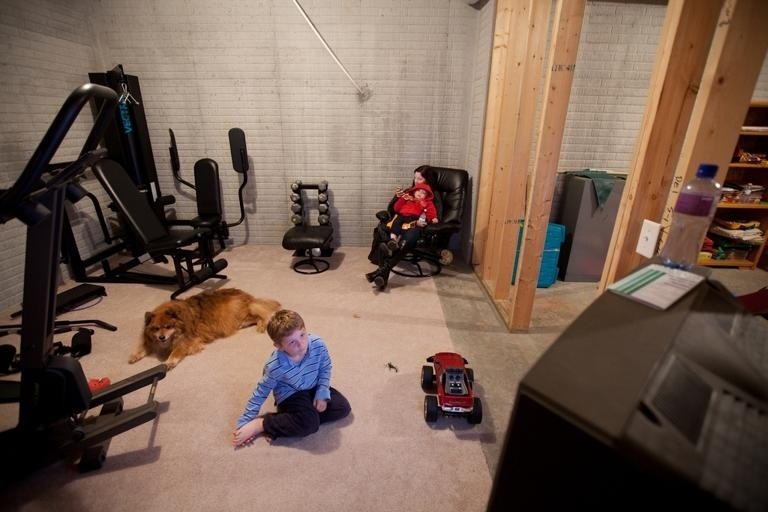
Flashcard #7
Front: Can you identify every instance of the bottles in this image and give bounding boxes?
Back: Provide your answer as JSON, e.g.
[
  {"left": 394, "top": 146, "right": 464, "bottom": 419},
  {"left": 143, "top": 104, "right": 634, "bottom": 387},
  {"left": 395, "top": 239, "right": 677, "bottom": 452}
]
[{"left": 661, "top": 161, "right": 722, "bottom": 270}]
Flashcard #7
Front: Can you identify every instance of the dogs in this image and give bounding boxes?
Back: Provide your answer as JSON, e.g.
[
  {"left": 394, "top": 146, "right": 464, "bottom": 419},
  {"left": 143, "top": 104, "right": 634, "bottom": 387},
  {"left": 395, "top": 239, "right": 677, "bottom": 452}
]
[{"left": 128, "top": 286, "right": 282, "bottom": 371}]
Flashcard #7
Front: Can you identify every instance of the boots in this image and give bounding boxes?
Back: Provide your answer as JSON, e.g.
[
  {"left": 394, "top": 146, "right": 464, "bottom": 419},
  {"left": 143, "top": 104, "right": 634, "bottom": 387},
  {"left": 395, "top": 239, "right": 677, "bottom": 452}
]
[
  {"left": 365, "top": 261, "right": 392, "bottom": 283},
  {"left": 373, "top": 267, "right": 391, "bottom": 291}
]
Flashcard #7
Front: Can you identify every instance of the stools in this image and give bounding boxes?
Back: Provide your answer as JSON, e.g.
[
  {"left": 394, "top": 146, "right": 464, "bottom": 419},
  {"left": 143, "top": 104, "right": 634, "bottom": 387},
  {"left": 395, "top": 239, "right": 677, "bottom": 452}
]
[{"left": 279, "top": 225, "right": 336, "bottom": 276}]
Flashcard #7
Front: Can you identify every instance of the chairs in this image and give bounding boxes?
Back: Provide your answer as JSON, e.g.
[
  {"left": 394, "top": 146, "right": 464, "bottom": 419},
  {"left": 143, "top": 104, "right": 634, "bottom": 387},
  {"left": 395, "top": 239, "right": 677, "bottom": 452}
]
[{"left": 374, "top": 165, "right": 468, "bottom": 279}]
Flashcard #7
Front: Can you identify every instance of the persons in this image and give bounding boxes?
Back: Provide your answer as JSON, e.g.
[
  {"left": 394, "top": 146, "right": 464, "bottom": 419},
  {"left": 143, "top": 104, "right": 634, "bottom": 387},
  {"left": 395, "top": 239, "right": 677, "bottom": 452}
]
[
  {"left": 381, "top": 182, "right": 439, "bottom": 258},
  {"left": 366, "top": 165, "right": 443, "bottom": 291},
  {"left": 233, "top": 309, "right": 352, "bottom": 447}
]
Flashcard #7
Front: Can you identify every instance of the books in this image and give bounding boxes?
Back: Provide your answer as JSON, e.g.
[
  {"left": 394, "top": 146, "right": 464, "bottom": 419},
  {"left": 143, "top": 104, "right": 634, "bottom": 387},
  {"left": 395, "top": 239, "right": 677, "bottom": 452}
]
[
  {"left": 607, "top": 262, "right": 707, "bottom": 311},
  {"left": 700, "top": 181, "right": 766, "bottom": 260}
]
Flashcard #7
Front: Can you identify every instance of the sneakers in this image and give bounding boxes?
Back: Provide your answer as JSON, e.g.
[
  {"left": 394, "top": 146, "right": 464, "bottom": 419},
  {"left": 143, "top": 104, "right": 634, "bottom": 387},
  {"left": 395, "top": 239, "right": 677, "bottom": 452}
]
[
  {"left": 386, "top": 239, "right": 401, "bottom": 251},
  {"left": 379, "top": 242, "right": 393, "bottom": 258}
]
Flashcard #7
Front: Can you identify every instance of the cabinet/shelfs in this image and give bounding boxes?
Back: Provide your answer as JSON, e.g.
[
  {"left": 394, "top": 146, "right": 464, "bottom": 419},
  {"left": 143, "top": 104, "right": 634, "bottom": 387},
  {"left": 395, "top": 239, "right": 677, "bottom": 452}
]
[{"left": 695, "top": 94, "right": 766, "bottom": 270}]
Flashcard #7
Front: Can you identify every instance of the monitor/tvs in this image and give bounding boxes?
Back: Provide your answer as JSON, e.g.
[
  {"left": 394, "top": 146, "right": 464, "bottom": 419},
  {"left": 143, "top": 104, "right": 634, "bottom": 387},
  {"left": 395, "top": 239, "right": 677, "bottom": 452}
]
[{"left": 486, "top": 255, "right": 768, "bottom": 512}]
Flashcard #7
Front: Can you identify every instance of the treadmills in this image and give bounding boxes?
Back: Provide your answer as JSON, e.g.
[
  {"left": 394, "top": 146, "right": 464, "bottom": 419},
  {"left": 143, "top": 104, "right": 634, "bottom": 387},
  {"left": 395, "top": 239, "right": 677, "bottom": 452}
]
[{"left": 1, "top": 83, "right": 167, "bottom": 481}]
